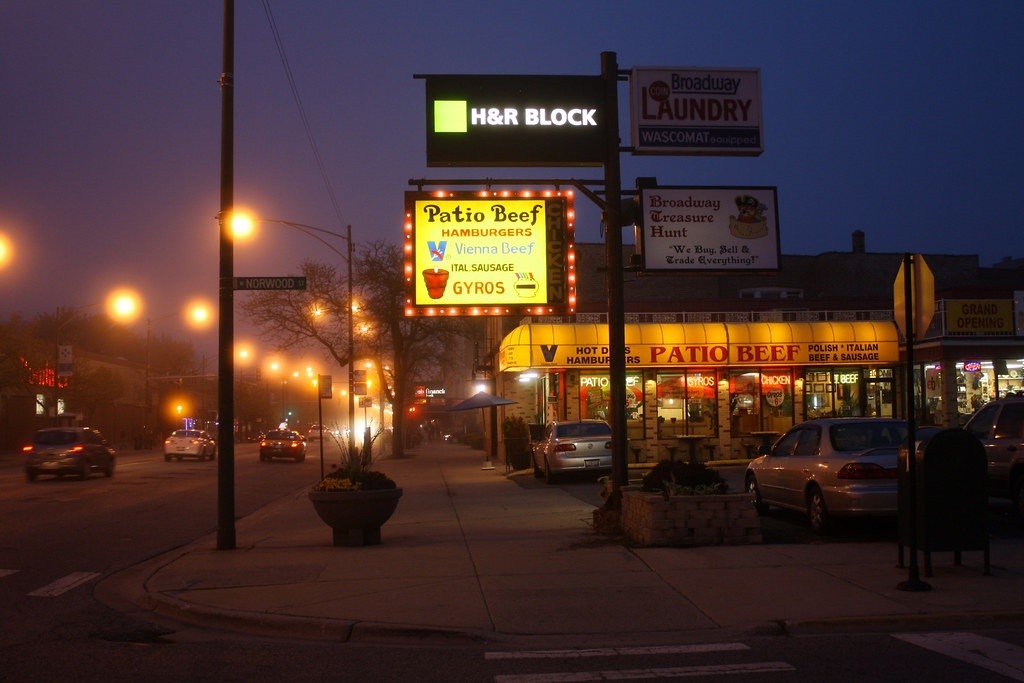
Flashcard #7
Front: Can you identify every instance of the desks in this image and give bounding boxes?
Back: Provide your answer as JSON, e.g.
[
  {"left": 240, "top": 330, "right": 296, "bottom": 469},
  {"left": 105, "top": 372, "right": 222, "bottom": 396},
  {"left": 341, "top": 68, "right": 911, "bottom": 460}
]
[
  {"left": 675, "top": 434, "right": 708, "bottom": 464},
  {"left": 751, "top": 431, "right": 779, "bottom": 456}
]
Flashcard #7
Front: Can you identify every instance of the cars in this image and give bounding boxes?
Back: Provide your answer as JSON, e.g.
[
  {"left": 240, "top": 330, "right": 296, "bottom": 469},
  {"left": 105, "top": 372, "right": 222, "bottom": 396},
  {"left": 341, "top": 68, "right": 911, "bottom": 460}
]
[
  {"left": 259, "top": 430, "right": 307, "bottom": 463},
  {"left": 744, "top": 419, "right": 917, "bottom": 529},
  {"left": 163, "top": 429, "right": 217, "bottom": 462},
  {"left": 962, "top": 395, "right": 1023, "bottom": 492},
  {"left": 308, "top": 425, "right": 332, "bottom": 442},
  {"left": 531, "top": 420, "right": 612, "bottom": 485},
  {"left": 23, "top": 426, "right": 116, "bottom": 482}
]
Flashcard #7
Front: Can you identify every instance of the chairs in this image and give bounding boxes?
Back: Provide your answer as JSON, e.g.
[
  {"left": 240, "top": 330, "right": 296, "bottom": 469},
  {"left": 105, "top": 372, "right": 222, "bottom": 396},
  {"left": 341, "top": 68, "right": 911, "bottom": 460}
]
[{"left": 872, "top": 436, "right": 889, "bottom": 449}]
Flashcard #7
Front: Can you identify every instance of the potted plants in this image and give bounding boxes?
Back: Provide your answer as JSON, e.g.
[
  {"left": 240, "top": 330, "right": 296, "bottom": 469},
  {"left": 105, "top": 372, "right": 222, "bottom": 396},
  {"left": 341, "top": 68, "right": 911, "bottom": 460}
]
[{"left": 502, "top": 415, "right": 532, "bottom": 470}]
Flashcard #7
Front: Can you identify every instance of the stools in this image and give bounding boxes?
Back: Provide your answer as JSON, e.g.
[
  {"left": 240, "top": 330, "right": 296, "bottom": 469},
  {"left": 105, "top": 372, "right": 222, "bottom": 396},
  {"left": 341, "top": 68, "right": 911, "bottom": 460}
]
[
  {"left": 667, "top": 446, "right": 678, "bottom": 463},
  {"left": 704, "top": 444, "right": 717, "bottom": 461},
  {"left": 632, "top": 447, "right": 642, "bottom": 462},
  {"left": 744, "top": 443, "right": 756, "bottom": 460}
]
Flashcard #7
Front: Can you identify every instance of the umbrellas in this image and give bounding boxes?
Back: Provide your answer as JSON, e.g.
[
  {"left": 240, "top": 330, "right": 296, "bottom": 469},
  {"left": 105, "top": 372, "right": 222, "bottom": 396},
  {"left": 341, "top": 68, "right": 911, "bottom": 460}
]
[{"left": 446, "top": 391, "right": 519, "bottom": 460}]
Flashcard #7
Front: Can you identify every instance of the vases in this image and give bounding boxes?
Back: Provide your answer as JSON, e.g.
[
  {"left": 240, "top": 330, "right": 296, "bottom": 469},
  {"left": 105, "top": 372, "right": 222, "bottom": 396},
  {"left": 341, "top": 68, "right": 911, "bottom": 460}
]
[{"left": 308, "top": 487, "right": 402, "bottom": 546}]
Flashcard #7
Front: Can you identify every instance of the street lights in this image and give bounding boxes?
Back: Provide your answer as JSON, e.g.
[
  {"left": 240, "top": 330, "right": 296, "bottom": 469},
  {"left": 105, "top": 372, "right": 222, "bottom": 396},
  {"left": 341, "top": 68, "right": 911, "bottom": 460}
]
[
  {"left": 54, "top": 295, "right": 135, "bottom": 416},
  {"left": 146, "top": 304, "right": 207, "bottom": 423},
  {"left": 229, "top": 212, "right": 357, "bottom": 465}
]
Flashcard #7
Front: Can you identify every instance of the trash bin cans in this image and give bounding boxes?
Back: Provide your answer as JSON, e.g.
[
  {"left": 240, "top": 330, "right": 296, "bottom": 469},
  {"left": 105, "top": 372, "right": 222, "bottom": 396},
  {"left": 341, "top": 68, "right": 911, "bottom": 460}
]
[{"left": 896, "top": 425, "right": 991, "bottom": 575}]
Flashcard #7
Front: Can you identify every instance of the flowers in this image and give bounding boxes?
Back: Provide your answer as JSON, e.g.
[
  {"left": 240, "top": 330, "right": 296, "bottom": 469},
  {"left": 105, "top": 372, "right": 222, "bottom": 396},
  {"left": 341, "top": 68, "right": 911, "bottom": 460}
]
[{"left": 311, "top": 423, "right": 398, "bottom": 491}]
